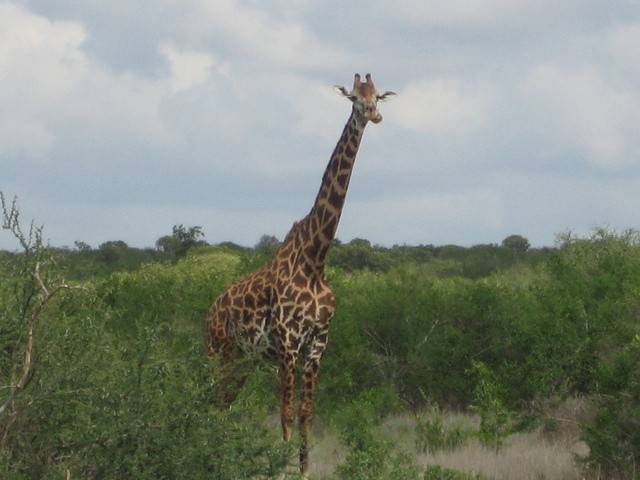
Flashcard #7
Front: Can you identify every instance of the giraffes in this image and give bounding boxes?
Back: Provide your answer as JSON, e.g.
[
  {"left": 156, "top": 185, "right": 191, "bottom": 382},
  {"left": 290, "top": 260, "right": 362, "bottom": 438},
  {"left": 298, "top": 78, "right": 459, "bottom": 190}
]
[{"left": 205, "top": 74, "right": 397, "bottom": 480}]
